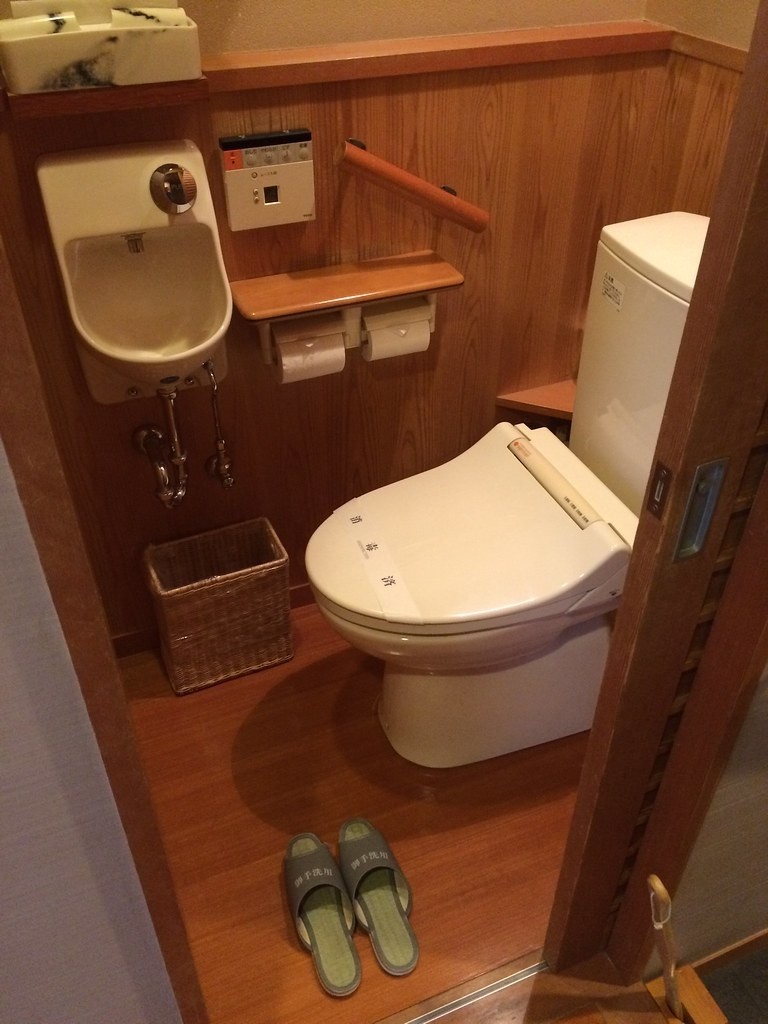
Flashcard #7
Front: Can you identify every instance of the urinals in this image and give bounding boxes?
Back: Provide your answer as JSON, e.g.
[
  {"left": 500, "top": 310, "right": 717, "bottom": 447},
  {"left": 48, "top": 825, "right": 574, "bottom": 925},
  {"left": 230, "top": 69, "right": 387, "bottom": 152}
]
[{"left": 36, "top": 136, "right": 237, "bottom": 406}]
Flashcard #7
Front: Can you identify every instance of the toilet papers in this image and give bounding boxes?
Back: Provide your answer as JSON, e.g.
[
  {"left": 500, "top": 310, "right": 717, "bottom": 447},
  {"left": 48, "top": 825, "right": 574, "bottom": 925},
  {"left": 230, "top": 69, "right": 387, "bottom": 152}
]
[
  {"left": 360, "top": 320, "right": 432, "bottom": 363},
  {"left": 269, "top": 333, "right": 346, "bottom": 384}
]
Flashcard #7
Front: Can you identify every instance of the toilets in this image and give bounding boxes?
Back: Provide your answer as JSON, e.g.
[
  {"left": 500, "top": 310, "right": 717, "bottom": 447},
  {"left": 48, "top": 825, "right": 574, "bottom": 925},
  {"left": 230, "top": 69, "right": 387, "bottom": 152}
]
[{"left": 304, "top": 207, "right": 762, "bottom": 771}]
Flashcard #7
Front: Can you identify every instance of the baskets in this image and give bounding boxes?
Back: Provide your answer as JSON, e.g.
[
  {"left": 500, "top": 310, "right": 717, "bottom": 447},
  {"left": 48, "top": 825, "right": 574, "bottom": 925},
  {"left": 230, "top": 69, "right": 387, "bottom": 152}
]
[{"left": 142, "top": 516, "right": 292, "bottom": 696}]
